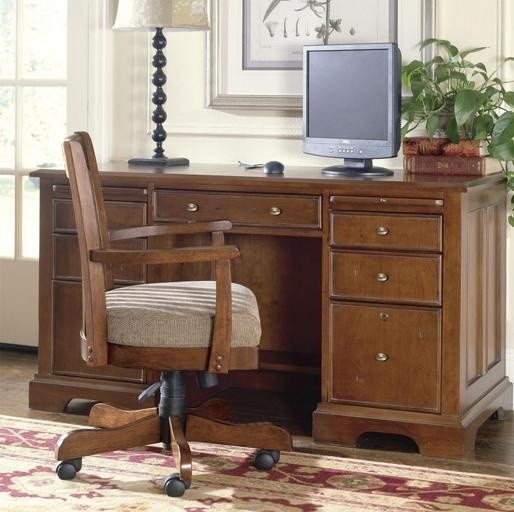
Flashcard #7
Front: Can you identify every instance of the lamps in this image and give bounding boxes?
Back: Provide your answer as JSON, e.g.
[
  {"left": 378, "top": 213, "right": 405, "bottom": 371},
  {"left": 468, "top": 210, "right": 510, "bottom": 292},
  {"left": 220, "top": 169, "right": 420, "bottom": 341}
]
[{"left": 113, "top": 0, "right": 210, "bottom": 167}]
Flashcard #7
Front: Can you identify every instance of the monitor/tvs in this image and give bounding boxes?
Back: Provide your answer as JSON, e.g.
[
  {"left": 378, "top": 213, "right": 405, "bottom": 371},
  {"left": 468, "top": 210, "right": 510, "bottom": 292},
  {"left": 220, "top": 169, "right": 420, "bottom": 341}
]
[{"left": 302, "top": 42, "right": 402, "bottom": 177}]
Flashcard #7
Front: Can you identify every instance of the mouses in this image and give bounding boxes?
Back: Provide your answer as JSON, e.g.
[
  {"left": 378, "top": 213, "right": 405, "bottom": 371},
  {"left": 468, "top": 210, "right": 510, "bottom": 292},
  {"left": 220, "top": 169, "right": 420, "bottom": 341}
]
[{"left": 263, "top": 160, "right": 285, "bottom": 174}]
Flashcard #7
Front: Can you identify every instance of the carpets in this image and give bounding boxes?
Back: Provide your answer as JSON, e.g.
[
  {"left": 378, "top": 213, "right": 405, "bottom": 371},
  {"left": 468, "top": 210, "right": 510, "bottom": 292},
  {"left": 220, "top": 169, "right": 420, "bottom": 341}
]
[{"left": 0, "top": 415, "right": 512, "bottom": 512}]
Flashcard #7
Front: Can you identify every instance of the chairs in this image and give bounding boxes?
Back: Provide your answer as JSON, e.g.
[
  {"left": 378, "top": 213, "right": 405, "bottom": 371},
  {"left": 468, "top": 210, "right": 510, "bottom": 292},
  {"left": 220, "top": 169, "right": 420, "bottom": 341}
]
[{"left": 56, "top": 133, "right": 291, "bottom": 497}]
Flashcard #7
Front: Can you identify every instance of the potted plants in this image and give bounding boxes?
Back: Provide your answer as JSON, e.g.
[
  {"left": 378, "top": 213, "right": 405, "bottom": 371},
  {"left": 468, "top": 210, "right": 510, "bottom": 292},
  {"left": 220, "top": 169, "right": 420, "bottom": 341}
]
[{"left": 399, "top": 40, "right": 514, "bottom": 228}]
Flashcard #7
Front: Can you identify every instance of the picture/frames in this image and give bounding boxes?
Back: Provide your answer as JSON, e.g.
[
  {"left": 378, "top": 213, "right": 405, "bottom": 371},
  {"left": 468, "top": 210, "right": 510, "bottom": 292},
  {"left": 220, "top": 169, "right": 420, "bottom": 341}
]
[{"left": 204, "top": 0, "right": 434, "bottom": 109}]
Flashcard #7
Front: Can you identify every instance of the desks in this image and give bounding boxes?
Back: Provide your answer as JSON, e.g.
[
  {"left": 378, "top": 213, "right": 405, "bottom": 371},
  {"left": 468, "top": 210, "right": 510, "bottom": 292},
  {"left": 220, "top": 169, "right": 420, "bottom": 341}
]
[{"left": 27, "top": 165, "right": 514, "bottom": 456}]
